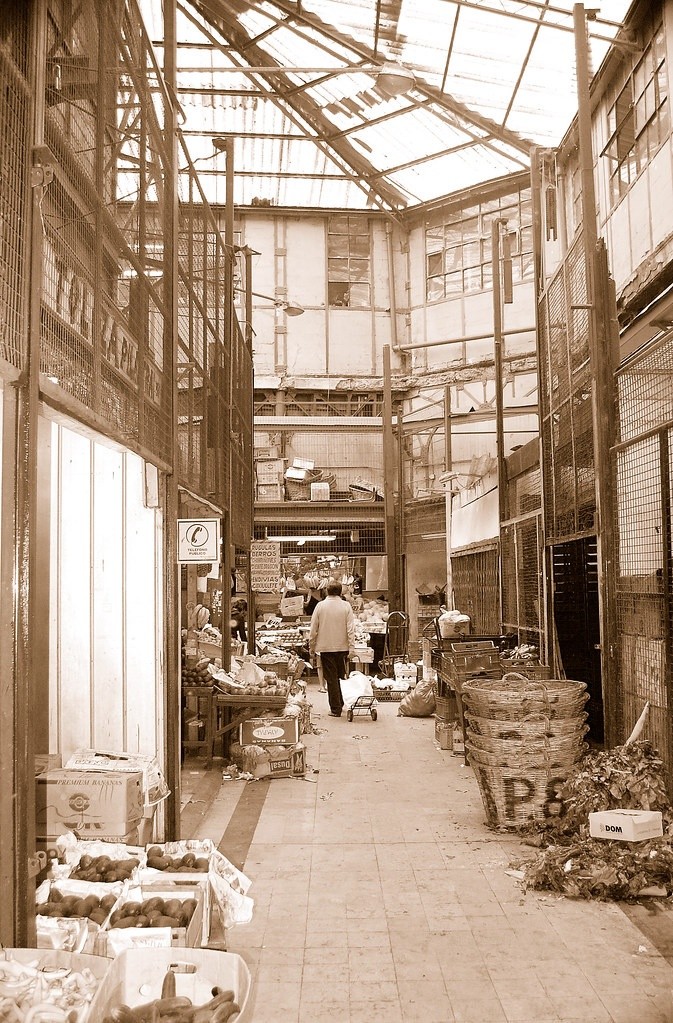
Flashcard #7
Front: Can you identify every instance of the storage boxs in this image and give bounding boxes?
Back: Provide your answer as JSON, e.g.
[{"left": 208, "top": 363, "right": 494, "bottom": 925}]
[
  {"left": 499, "top": 657, "right": 550, "bottom": 681},
  {"left": 0, "top": 714, "right": 307, "bottom": 1023},
  {"left": 451, "top": 641, "right": 501, "bottom": 671},
  {"left": 589, "top": 809, "right": 663, "bottom": 841}
]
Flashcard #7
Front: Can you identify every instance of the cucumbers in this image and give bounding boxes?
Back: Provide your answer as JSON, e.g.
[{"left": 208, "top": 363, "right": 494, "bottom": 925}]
[{"left": 104, "top": 970, "right": 243, "bottom": 1023}]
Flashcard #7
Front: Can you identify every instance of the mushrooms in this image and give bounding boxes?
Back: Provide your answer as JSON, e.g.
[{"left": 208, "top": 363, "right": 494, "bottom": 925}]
[{"left": 0, "top": 947, "right": 98, "bottom": 1023}]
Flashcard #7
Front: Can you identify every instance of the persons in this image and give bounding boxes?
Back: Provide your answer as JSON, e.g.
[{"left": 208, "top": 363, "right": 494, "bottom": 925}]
[
  {"left": 219, "top": 600, "right": 247, "bottom": 642},
  {"left": 308, "top": 581, "right": 355, "bottom": 717}
]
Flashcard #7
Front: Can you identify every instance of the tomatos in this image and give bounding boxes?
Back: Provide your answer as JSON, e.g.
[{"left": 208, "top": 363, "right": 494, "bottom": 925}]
[
  {"left": 254, "top": 633, "right": 305, "bottom": 643},
  {"left": 182, "top": 662, "right": 214, "bottom": 688},
  {"left": 219, "top": 674, "right": 287, "bottom": 696}
]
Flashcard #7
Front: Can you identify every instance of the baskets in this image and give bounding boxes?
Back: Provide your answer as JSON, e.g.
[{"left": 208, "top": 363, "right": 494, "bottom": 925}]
[{"left": 460, "top": 671, "right": 591, "bottom": 828}]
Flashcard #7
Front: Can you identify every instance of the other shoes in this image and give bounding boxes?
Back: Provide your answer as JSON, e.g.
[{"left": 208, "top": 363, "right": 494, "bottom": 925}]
[{"left": 328, "top": 712, "right": 341, "bottom": 717}]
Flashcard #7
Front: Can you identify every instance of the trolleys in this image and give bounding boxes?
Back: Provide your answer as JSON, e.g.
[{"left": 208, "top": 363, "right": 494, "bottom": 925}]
[{"left": 343, "top": 654, "right": 378, "bottom": 722}]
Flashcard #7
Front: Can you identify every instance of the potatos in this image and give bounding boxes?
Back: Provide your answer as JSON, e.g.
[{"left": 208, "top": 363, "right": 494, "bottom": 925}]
[
  {"left": 68, "top": 854, "right": 140, "bottom": 883},
  {"left": 36, "top": 887, "right": 117, "bottom": 927},
  {"left": 146, "top": 846, "right": 209, "bottom": 885},
  {"left": 106, "top": 897, "right": 198, "bottom": 928}
]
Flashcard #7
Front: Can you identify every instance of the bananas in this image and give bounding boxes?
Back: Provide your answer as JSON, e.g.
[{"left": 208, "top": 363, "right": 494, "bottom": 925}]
[
  {"left": 190, "top": 604, "right": 210, "bottom": 628},
  {"left": 308, "top": 575, "right": 334, "bottom": 590}
]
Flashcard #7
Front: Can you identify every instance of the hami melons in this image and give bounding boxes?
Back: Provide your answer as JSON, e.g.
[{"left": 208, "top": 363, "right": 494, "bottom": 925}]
[{"left": 357, "top": 601, "right": 389, "bottom": 622}]
